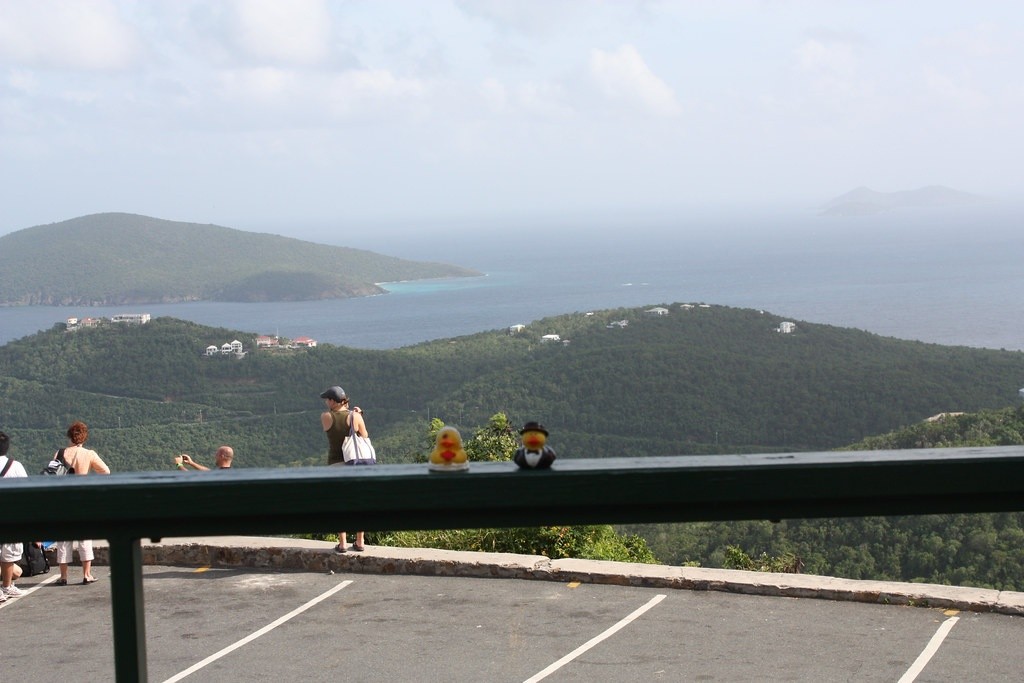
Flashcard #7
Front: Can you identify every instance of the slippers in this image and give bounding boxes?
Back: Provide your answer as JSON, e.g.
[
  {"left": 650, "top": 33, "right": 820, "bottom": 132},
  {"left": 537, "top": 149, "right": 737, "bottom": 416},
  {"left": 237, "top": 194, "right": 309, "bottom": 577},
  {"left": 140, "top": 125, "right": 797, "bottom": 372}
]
[
  {"left": 335, "top": 543, "right": 347, "bottom": 552},
  {"left": 353, "top": 541, "right": 364, "bottom": 551}
]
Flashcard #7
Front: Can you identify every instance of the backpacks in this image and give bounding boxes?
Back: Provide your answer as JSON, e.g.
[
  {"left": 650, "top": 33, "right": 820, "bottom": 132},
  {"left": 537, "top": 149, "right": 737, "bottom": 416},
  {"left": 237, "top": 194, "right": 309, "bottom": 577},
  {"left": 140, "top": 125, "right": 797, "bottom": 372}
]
[
  {"left": 43, "top": 448, "right": 75, "bottom": 475},
  {"left": 25, "top": 543, "right": 50, "bottom": 574}
]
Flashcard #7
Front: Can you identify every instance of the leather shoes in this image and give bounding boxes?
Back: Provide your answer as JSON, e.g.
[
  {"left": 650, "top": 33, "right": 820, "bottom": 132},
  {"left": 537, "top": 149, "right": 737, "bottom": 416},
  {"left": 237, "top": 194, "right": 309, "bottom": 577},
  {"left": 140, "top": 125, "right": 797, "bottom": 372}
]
[
  {"left": 83, "top": 576, "right": 99, "bottom": 586},
  {"left": 56, "top": 578, "right": 68, "bottom": 585}
]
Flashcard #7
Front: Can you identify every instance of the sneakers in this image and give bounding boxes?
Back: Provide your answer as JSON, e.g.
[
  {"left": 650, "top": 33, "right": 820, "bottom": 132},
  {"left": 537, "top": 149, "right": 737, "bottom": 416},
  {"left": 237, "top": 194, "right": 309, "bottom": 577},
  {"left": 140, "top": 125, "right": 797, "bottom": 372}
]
[
  {"left": 0, "top": 590, "right": 6, "bottom": 601},
  {"left": 1, "top": 582, "right": 24, "bottom": 597}
]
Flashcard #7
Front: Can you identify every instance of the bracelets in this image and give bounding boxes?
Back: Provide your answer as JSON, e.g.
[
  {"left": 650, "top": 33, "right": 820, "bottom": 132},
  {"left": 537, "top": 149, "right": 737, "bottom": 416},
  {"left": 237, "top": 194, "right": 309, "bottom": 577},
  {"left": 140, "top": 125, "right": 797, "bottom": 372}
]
[{"left": 176, "top": 463, "right": 184, "bottom": 468}]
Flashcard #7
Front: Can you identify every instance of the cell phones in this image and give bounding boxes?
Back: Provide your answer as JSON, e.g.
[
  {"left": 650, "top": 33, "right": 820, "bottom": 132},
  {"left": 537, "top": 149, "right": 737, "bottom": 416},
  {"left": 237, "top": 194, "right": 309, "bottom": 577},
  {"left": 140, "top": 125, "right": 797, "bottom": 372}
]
[{"left": 183, "top": 456, "right": 187, "bottom": 460}]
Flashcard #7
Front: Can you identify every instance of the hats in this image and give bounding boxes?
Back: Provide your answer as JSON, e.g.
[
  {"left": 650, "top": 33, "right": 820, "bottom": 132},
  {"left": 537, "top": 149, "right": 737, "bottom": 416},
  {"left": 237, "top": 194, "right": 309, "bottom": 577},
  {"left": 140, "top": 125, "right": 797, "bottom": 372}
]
[{"left": 320, "top": 386, "right": 347, "bottom": 402}]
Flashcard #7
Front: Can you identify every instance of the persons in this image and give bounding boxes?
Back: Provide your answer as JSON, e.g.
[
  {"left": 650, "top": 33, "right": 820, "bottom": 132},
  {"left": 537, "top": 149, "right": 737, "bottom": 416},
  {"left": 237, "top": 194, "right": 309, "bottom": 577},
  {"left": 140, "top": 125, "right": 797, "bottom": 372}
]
[
  {"left": 0, "top": 431, "right": 42, "bottom": 602},
  {"left": 174, "top": 446, "right": 233, "bottom": 471},
  {"left": 51, "top": 422, "right": 111, "bottom": 584},
  {"left": 320, "top": 387, "right": 369, "bottom": 553}
]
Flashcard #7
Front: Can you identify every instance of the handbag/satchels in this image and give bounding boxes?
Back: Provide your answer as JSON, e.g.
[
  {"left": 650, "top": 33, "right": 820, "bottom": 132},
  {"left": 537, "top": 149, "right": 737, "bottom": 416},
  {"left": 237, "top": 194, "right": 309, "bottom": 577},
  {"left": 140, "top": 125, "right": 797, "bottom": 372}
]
[{"left": 341, "top": 411, "right": 376, "bottom": 464}]
